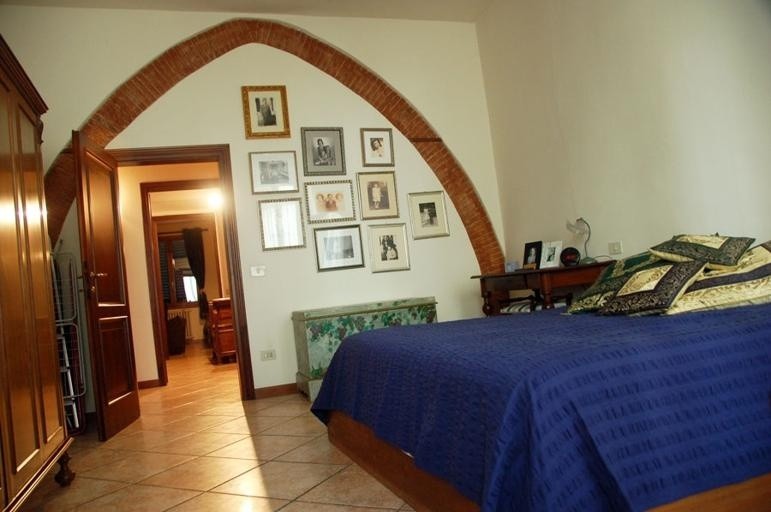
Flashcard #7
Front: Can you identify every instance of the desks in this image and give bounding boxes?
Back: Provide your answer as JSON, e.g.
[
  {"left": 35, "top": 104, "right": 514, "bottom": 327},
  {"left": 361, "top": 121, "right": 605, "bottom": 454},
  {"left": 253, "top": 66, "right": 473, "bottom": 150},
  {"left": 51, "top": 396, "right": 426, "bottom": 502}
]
[{"left": 471, "top": 260, "right": 616, "bottom": 316}]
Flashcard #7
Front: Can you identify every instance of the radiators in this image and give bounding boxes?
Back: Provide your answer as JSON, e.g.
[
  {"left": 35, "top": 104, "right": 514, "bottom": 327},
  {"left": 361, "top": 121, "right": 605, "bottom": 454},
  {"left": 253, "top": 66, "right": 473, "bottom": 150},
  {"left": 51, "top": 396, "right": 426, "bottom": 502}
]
[{"left": 168, "top": 309, "right": 196, "bottom": 340}]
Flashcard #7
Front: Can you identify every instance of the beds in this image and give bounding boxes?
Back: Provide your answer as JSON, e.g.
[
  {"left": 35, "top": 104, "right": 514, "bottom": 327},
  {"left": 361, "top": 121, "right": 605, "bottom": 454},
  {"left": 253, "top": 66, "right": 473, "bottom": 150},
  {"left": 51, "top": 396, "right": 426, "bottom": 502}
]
[{"left": 310, "top": 304, "right": 770, "bottom": 512}]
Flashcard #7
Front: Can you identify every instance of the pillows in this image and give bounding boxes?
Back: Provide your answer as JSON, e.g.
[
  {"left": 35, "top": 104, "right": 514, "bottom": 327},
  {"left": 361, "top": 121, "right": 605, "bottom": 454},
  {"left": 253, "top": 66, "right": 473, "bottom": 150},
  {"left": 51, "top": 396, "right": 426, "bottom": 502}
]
[
  {"left": 597, "top": 259, "right": 708, "bottom": 315},
  {"left": 567, "top": 251, "right": 661, "bottom": 313},
  {"left": 661, "top": 241, "right": 770, "bottom": 316},
  {"left": 647, "top": 233, "right": 755, "bottom": 269}
]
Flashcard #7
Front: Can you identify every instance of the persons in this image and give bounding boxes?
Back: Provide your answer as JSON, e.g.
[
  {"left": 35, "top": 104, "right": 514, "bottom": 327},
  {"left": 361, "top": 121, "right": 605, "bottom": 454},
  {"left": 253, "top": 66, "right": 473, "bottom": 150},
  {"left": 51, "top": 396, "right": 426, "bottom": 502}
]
[
  {"left": 260, "top": 98, "right": 275, "bottom": 125},
  {"left": 317, "top": 192, "right": 344, "bottom": 211},
  {"left": 372, "top": 183, "right": 384, "bottom": 209},
  {"left": 528, "top": 247, "right": 535, "bottom": 264},
  {"left": 317, "top": 139, "right": 333, "bottom": 165},
  {"left": 380, "top": 235, "right": 398, "bottom": 260},
  {"left": 371, "top": 139, "right": 383, "bottom": 157}
]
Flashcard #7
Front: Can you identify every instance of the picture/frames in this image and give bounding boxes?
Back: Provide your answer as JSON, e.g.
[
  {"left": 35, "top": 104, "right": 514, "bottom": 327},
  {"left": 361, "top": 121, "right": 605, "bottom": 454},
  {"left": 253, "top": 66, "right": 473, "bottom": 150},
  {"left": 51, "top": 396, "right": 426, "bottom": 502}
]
[
  {"left": 301, "top": 126, "right": 346, "bottom": 177},
  {"left": 259, "top": 197, "right": 306, "bottom": 251},
  {"left": 304, "top": 180, "right": 356, "bottom": 224},
  {"left": 408, "top": 190, "right": 449, "bottom": 240},
  {"left": 367, "top": 223, "right": 409, "bottom": 273},
  {"left": 539, "top": 241, "right": 562, "bottom": 268},
  {"left": 241, "top": 85, "right": 290, "bottom": 139},
  {"left": 313, "top": 224, "right": 364, "bottom": 273},
  {"left": 359, "top": 128, "right": 394, "bottom": 167},
  {"left": 250, "top": 151, "right": 299, "bottom": 194},
  {"left": 355, "top": 171, "right": 399, "bottom": 220},
  {"left": 523, "top": 241, "right": 542, "bottom": 268}
]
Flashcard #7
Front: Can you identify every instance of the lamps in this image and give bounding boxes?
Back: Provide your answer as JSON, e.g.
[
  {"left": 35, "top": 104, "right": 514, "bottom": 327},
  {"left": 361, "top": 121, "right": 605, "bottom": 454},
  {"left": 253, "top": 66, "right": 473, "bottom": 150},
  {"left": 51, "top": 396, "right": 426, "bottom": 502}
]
[{"left": 573, "top": 217, "right": 596, "bottom": 264}]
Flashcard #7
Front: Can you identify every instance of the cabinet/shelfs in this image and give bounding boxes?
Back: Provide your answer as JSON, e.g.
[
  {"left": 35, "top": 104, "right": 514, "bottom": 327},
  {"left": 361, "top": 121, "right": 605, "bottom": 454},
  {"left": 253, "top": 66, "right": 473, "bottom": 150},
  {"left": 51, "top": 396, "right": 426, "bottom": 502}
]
[
  {"left": 209, "top": 297, "right": 237, "bottom": 362},
  {"left": 0, "top": 34, "right": 73, "bottom": 512}
]
